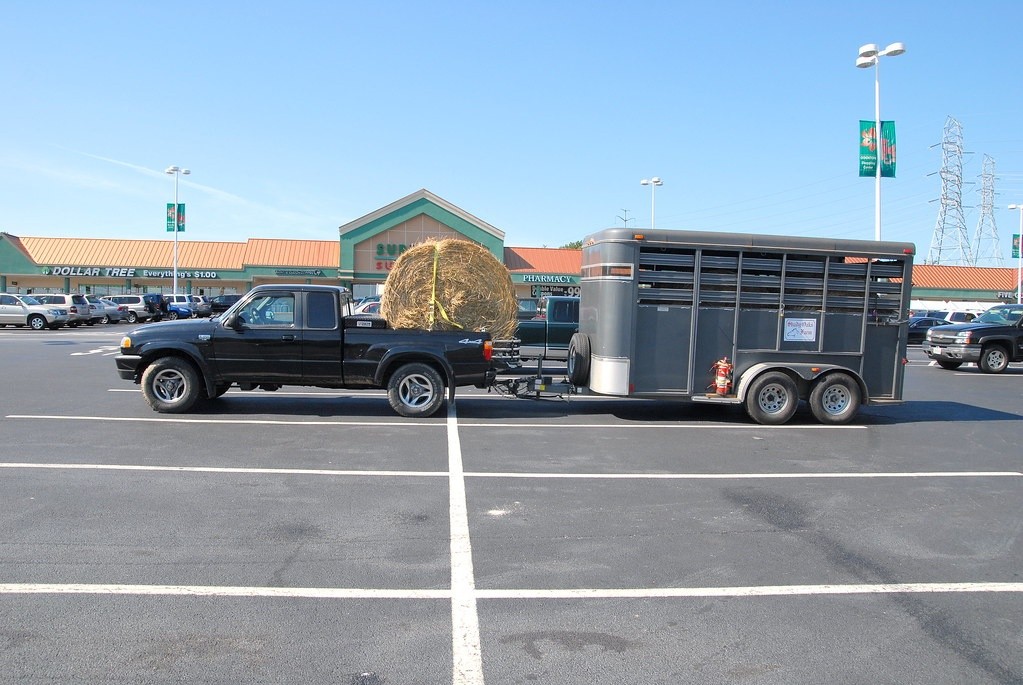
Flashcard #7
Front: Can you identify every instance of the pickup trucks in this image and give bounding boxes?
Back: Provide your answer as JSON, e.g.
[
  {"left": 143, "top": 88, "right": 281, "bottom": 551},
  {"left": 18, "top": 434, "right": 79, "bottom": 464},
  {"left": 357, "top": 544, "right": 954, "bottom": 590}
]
[
  {"left": 513, "top": 295, "right": 580, "bottom": 363},
  {"left": 114, "top": 282, "right": 498, "bottom": 416}
]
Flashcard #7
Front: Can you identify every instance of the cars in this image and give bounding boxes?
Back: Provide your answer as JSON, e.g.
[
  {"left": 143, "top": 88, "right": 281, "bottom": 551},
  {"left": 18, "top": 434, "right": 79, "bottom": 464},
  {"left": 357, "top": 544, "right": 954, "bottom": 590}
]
[
  {"left": 346, "top": 292, "right": 383, "bottom": 317},
  {"left": 908, "top": 308, "right": 987, "bottom": 325},
  {"left": 906, "top": 316, "right": 954, "bottom": 344},
  {"left": 100, "top": 294, "right": 275, "bottom": 323},
  {"left": 26, "top": 293, "right": 92, "bottom": 328},
  {"left": 100, "top": 298, "right": 128, "bottom": 324},
  {"left": 0, "top": 293, "right": 69, "bottom": 330},
  {"left": 83, "top": 293, "right": 107, "bottom": 325}
]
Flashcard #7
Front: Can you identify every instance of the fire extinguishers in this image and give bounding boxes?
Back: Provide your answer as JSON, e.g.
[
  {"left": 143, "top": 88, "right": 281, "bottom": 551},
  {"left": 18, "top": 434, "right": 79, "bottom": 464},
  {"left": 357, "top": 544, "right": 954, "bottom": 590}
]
[{"left": 715, "top": 360, "right": 729, "bottom": 396}]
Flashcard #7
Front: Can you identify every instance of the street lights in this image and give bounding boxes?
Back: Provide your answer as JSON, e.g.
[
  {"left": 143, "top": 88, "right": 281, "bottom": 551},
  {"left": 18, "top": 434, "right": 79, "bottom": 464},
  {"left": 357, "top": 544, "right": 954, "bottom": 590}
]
[
  {"left": 854, "top": 41, "right": 907, "bottom": 237},
  {"left": 640, "top": 177, "right": 664, "bottom": 230},
  {"left": 166, "top": 165, "right": 190, "bottom": 306},
  {"left": 1008, "top": 204, "right": 1023, "bottom": 304}
]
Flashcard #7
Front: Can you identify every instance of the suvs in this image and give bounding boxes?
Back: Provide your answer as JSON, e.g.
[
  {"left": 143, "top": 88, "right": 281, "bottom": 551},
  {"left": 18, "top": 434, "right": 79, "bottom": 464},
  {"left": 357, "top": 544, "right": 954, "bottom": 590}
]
[{"left": 921, "top": 304, "right": 1023, "bottom": 373}]
